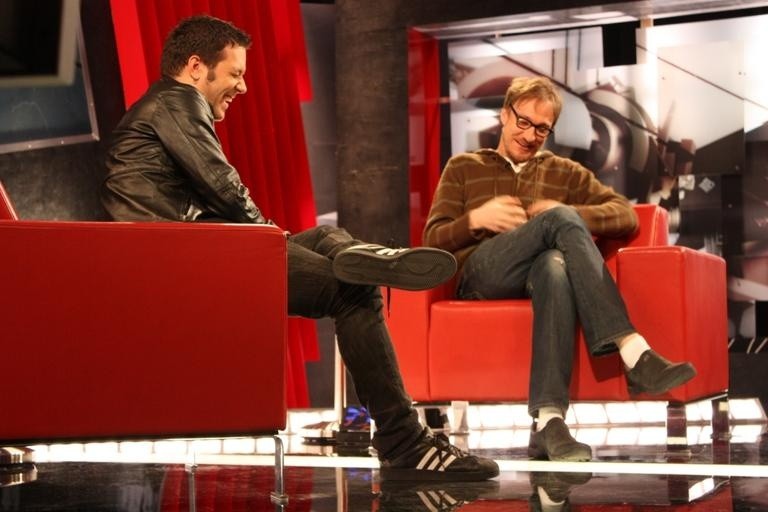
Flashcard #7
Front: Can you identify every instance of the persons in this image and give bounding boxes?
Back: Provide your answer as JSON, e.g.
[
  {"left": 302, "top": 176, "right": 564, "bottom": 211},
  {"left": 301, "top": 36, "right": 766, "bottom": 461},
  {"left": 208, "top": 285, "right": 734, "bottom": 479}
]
[
  {"left": 423, "top": 77, "right": 696, "bottom": 461},
  {"left": 84, "top": 15, "right": 499, "bottom": 481}
]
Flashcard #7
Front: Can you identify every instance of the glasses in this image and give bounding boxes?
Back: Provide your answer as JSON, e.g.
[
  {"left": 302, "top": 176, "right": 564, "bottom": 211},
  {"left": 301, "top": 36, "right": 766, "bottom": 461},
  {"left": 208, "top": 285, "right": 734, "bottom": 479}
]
[{"left": 506, "top": 96, "right": 557, "bottom": 140}]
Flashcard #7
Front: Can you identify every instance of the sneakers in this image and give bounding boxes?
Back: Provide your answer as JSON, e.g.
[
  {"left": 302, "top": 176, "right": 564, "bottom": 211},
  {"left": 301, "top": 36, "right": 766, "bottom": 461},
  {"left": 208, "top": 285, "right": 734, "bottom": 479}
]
[
  {"left": 375, "top": 481, "right": 501, "bottom": 512},
  {"left": 375, "top": 423, "right": 499, "bottom": 482},
  {"left": 330, "top": 238, "right": 461, "bottom": 291}
]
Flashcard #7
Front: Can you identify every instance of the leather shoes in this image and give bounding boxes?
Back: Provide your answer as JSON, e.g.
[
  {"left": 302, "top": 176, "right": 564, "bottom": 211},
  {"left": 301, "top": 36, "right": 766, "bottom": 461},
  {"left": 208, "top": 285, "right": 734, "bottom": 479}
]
[
  {"left": 525, "top": 413, "right": 595, "bottom": 466},
  {"left": 620, "top": 344, "right": 699, "bottom": 402},
  {"left": 529, "top": 471, "right": 594, "bottom": 507}
]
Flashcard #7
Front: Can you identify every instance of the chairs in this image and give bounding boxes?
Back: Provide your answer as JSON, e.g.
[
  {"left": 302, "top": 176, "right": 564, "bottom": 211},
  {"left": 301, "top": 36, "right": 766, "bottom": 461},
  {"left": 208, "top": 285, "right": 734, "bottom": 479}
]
[
  {"left": 0, "top": 179, "right": 296, "bottom": 508},
  {"left": 375, "top": 200, "right": 733, "bottom": 451}
]
